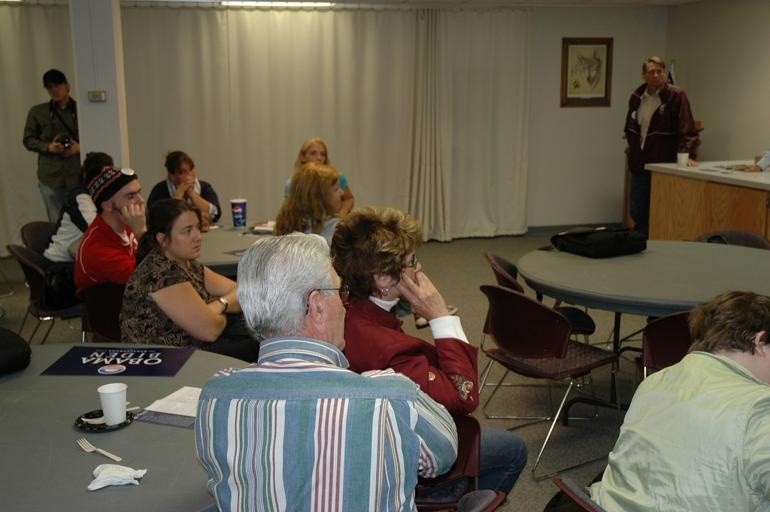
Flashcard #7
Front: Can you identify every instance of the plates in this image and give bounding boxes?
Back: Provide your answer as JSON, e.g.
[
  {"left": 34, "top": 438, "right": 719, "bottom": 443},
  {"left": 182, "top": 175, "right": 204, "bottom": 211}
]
[
  {"left": 74, "top": 409, "right": 133, "bottom": 433},
  {"left": 199, "top": 224, "right": 224, "bottom": 231}
]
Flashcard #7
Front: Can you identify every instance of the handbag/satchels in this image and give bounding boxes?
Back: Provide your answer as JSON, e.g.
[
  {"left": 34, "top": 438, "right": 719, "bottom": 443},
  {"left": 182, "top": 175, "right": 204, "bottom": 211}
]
[{"left": 550, "top": 223, "right": 648, "bottom": 260}]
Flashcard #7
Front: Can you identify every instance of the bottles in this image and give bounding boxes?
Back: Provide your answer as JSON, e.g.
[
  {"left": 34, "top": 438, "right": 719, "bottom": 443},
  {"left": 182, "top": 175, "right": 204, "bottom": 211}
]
[{"left": 62, "top": 137, "right": 71, "bottom": 155}]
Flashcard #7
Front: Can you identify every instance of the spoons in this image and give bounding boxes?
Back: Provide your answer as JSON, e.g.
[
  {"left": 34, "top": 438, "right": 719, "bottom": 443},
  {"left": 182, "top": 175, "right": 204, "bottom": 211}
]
[{"left": 88, "top": 406, "right": 140, "bottom": 424}]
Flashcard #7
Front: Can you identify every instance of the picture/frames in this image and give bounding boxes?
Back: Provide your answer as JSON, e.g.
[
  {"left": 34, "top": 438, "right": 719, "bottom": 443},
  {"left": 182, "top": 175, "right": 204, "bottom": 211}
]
[{"left": 560, "top": 36, "right": 613, "bottom": 107}]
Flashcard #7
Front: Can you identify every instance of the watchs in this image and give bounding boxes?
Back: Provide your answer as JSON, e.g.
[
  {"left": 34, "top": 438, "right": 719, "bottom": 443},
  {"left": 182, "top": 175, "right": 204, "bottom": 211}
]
[{"left": 218, "top": 296, "right": 229, "bottom": 314}]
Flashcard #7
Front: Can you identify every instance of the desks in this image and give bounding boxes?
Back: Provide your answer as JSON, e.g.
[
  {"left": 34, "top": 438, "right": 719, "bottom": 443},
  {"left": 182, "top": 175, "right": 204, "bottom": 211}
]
[
  {"left": 643, "top": 158, "right": 769, "bottom": 241},
  {"left": 1, "top": 341, "right": 258, "bottom": 512},
  {"left": 193, "top": 225, "right": 273, "bottom": 267},
  {"left": 517, "top": 238, "right": 769, "bottom": 426}
]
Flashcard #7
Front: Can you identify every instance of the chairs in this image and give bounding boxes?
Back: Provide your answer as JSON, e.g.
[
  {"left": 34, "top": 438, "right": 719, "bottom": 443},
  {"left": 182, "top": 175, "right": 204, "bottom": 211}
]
[
  {"left": 479, "top": 282, "right": 621, "bottom": 482},
  {"left": 415, "top": 415, "right": 479, "bottom": 502},
  {"left": 417, "top": 488, "right": 504, "bottom": 512},
  {"left": 642, "top": 230, "right": 769, "bottom": 379},
  {"left": 553, "top": 476, "right": 607, "bottom": 512},
  {"left": 6, "top": 222, "right": 90, "bottom": 343},
  {"left": 479, "top": 250, "right": 597, "bottom": 392}
]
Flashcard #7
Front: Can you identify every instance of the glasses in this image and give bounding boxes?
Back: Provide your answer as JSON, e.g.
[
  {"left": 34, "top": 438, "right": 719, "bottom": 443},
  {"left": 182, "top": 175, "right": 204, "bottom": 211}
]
[
  {"left": 406, "top": 254, "right": 418, "bottom": 267},
  {"left": 305, "top": 284, "right": 350, "bottom": 316}
]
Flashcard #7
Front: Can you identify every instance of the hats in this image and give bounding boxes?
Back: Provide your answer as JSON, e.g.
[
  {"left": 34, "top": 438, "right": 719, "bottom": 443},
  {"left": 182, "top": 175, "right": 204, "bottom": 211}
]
[
  {"left": 43, "top": 69, "right": 65, "bottom": 87},
  {"left": 86, "top": 165, "right": 137, "bottom": 209}
]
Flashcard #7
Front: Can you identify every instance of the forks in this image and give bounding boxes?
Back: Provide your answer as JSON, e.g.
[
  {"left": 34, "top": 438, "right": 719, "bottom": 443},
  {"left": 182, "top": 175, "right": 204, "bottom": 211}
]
[{"left": 77, "top": 438, "right": 122, "bottom": 462}]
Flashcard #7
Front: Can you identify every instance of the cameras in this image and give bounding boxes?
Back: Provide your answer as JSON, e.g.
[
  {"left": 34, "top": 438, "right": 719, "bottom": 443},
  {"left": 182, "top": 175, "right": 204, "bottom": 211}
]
[{"left": 58, "top": 135, "right": 71, "bottom": 149}]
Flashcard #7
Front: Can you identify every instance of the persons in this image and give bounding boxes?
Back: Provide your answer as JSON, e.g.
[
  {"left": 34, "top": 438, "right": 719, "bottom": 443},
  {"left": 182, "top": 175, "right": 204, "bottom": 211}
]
[
  {"left": 145, "top": 148, "right": 223, "bottom": 234},
  {"left": 623, "top": 55, "right": 702, "bottom": 239},
  {"left": 116, "top": 196, "right": 259, "bottom": 364},
  {"left": 73, "top": 164, "right": 149, "bottom": 299},
  {"left": 282, "top": 136, "right": 356, "bottom": 218},
  {"left": 272, "top": 159, "right": 345, "bottom": 246},
  {"left": 585, "top": 290, "right": 769, "bottom": 512},
  {"left": 194, "top": 230, "right": 460, "bottom": 512},
  {"left": 328, "top": 205, "right": 530, "bottom": 508},
  {"left": 22, "top": 68, "right": 82, "bottom": 223},
  {"left": 32, "top": 149, "right": 115, "bottom": 264}
]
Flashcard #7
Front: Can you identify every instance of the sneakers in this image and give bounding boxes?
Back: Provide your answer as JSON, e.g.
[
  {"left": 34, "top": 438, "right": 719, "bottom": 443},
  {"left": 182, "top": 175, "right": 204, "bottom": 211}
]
[{"left": 415, "top": 307, "right": 457, "bottom": 328}]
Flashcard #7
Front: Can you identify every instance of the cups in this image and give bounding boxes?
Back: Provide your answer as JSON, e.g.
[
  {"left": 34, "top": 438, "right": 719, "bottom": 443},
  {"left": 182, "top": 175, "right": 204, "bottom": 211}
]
[
  {"left": 97, "top": 383, "right": 129, "bottom": 426},
  {"left": 676, "top": 148, "right": 689, "bottom": 170},
  {"left": 230, "top": 198, "right": 247, "bottom": 230}
]
